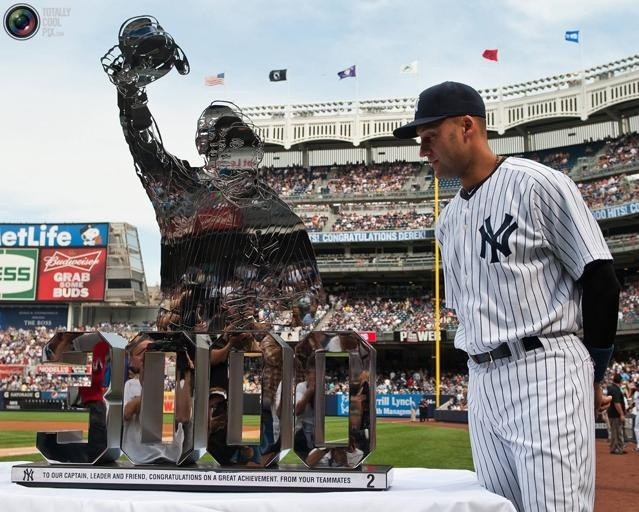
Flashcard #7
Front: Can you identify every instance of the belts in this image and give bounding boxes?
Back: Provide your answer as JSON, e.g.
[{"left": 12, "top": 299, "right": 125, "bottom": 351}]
[{"left": 470, "top": 336, "right": 543, "bottom": 365}]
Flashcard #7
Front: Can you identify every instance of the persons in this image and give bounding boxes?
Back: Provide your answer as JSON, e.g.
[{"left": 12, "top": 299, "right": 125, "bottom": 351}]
[
  {"left": 395, "top": 81, "right": 622, "bottom": 511},
  {"left": 1, "top": 268, "right": 638, "bottom": 426},
  {"left": 306, "top": 436, "right": 365, "bottom": 469},
  {"left": 122, "top": 337, "right": 195, "bottom": 464},
  {"left": 259, "top": 131, "right": 639, "bottom": 271},
  {"left": 295, "top": 353, "right": 315, "bottom": 457},
  {"left": 629, "top": 391, "right": 638, "bottom": 451},
  {"left": 605, "top": 374, "right": 627, "bottom": 454}
]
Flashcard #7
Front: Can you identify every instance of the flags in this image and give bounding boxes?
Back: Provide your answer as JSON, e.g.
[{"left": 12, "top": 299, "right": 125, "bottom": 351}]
[
  {"left": 401, "top": 60, "right": 420, "bottom": 74},
  {"left": 206, "top": 73, "right": 224, "bottom": 87},
  {"left": 482, "top": 50, "right": 498, "bottom": 62},
  {"left": 337, "top": 65, "right": 356, "bottom": 80},
  {"left": 269, "top": 69, "right": 287, "bottom": 81},
  {"left": 564, "top": 31, "right": 579, "bottom": 43}
]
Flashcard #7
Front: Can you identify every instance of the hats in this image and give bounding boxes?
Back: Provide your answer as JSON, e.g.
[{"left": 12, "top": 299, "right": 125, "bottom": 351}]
[{"left": 392, "top": 81, "right": 487, "bottom": 139}]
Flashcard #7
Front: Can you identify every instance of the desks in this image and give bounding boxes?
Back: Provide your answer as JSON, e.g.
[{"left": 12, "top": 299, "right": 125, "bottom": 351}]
[{"left": 0, "top": 461, "right": 516, "bottom": 512}]
